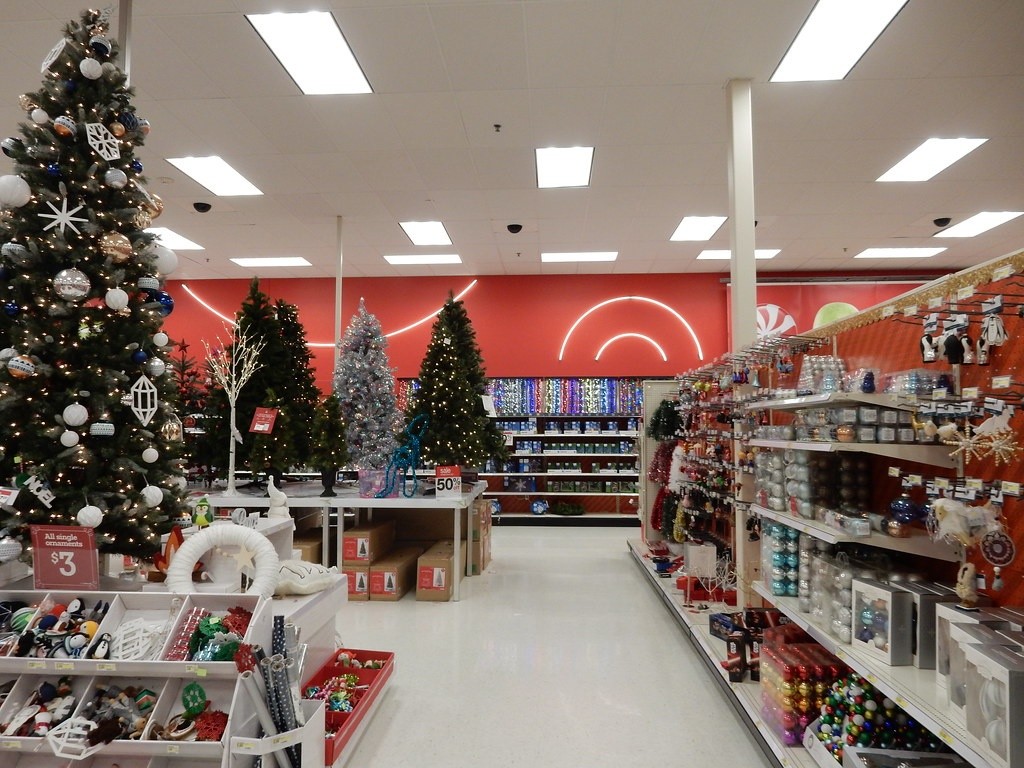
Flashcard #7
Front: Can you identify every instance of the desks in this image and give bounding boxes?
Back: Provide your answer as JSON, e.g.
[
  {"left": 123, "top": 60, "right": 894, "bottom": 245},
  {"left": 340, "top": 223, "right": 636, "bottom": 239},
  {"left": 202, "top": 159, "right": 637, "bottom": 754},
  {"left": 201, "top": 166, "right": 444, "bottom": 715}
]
[{"left": 185, "top": 479, "right": 489, "bottom": 602}]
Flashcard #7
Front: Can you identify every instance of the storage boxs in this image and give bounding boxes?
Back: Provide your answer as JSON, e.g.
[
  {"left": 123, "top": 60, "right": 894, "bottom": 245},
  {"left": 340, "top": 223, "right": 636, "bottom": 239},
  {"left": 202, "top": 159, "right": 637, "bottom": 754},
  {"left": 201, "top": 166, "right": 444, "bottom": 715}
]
[
  {"left": 342, "top": 499, "right": 491, "bottom": 601},
  {"left": 485, "top": 417, "right": 640, "bottom": 493},
  {"left": 677, "top": 542, "right": 737, "bottom": 606},
  {"left": 709, "top": 580, "right": 1024, "bottom": 768},
  {"left": 294, "top": 527, "right": 337, "bottom": 565}
]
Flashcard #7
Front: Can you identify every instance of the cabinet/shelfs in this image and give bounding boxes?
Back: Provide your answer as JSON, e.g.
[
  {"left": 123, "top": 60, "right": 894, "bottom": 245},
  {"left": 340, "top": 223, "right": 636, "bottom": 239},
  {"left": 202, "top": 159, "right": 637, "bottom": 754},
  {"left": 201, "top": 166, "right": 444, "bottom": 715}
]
[
  {"left": 689, "top": 250, "right": 1024, "bottom": 768},
  {"left": 234, "top": 375, "right": 677, "bottom": 518}
]
